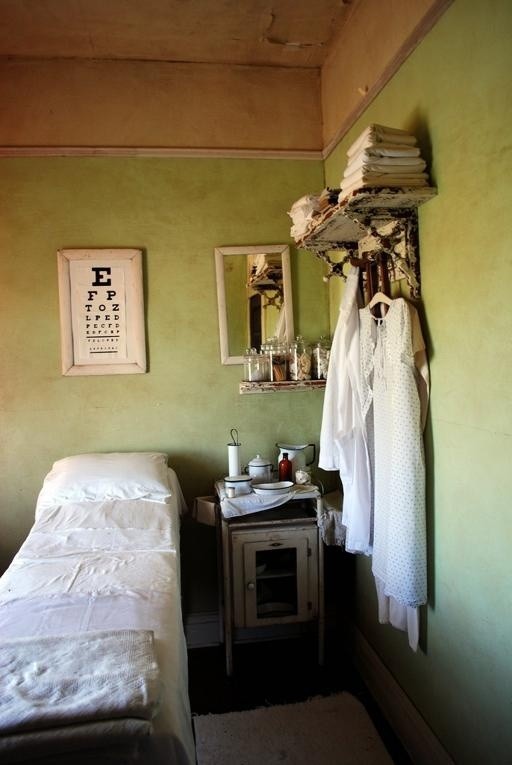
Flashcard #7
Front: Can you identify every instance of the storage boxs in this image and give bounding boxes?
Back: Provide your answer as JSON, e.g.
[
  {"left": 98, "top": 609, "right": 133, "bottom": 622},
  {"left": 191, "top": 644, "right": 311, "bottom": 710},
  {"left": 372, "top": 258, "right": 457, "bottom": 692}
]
[{"left": 186, "top": 609, "right": 219, "bottom": 646}]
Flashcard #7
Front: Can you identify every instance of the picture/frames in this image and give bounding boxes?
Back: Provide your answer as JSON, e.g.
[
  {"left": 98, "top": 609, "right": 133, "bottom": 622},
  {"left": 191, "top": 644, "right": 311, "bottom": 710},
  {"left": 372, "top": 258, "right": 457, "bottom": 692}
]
[{"left": 57, "top": 249, "right": 146, "bottom": 376}]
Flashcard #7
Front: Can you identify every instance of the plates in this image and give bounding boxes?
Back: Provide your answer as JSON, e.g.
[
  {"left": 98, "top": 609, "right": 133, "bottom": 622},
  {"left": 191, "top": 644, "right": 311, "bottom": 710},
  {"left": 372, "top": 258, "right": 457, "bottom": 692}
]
[{"left": 249, "top": 480, "right": 296, "bottom": 496}]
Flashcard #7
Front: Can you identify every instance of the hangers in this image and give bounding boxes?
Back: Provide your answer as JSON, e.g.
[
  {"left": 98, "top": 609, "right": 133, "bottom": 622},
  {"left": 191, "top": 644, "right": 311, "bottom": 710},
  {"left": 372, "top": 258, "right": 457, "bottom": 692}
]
[{"left": 322, "top": 253, "right": 434, "bottom": 338}]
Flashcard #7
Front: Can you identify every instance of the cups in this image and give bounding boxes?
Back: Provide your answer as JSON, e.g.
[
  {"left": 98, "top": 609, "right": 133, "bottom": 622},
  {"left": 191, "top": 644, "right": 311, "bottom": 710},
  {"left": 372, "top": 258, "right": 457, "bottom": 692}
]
[{"left": 244, "top": 454, "right": 275, "bottom": 485}]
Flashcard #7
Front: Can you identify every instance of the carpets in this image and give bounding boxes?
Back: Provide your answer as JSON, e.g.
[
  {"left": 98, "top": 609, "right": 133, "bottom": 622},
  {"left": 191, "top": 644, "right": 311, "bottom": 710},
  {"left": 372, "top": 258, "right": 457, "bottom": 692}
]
[{"left": 193, "top": 692, "right": 395, "bottom": 765}]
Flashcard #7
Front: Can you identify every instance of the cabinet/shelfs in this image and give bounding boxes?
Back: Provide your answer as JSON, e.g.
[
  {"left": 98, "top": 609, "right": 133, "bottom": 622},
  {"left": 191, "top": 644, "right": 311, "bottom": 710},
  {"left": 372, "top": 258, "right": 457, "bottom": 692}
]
[{"left": 215, "top": 480, "right": 324, "bottom": 642}]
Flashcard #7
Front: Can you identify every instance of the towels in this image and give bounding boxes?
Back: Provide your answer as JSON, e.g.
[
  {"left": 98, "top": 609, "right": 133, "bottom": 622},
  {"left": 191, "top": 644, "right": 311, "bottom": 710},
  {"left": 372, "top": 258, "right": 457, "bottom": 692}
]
[
  {"left": 0, "top": 629, "right": 163, "bottom": 731},
  {"left": 248, "top": 249, "right": 285, "bottom": 282},
  {"left": 339, "top": 124, "right": 430, "bottom": 197},
  {"left": 214, "top": 477, "right": 319, "bottom": 518},
  {"left": 289, "top": 181, "right": 333, "bottom": 237}
]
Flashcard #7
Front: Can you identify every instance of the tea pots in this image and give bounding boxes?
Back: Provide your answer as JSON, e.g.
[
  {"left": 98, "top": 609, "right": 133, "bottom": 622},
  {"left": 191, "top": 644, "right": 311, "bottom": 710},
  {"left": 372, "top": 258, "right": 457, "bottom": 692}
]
[{"left": 275, "top": 442, "right": 316, "bottom": 482}]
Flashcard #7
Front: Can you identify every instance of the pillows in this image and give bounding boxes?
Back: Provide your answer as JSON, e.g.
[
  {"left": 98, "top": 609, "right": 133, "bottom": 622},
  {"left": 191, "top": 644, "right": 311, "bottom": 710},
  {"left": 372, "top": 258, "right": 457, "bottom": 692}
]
[{"left": 42, "top": 453, "right": 172, "bottom": 506}]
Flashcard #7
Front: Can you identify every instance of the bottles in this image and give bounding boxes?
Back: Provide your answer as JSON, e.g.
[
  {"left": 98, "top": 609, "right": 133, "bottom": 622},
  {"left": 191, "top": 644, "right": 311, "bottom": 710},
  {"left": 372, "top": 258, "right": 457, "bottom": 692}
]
[
  {"left": 249, "top": 349, "right": 261, "bottom": 381},
  {"left": 289, "top": 335, "right": 313, "bottom": 382},
  {"left": 313, "top": 334, "right": 332, "bottom": 381},
  {"left": 260, "top": 335, "right": 288, "bottom": 381},
  {"left": 242, "top": 348, "right": 250, "bottom": 381},
  {"left": 278, "top": 453, "right": 293, "bottom": 482}
]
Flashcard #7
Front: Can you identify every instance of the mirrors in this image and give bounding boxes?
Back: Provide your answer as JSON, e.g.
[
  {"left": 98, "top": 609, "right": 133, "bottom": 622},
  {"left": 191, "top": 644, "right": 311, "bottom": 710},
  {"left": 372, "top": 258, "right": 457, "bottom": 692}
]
[{"left": 214, "top": 244, "right": 294, "bottom": 365}]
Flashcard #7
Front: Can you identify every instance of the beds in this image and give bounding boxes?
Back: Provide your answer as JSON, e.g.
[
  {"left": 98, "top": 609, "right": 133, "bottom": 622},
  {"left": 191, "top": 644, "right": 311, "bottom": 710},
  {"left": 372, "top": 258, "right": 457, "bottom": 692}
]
[{"left": 0, "top": 452, "right": 194, "bottom": 764}]
